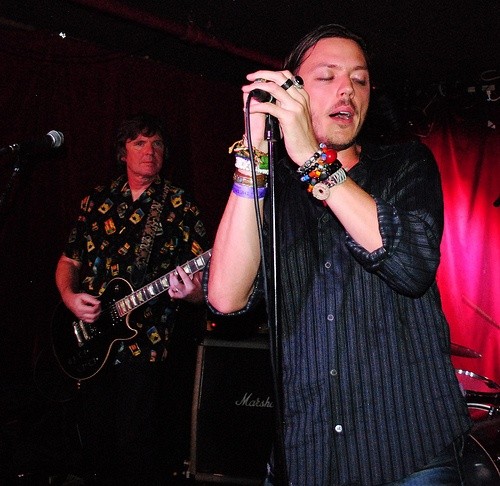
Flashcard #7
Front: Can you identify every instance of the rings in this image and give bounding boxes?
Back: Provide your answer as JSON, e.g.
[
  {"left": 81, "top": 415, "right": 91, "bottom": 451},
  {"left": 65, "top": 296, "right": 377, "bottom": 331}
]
[
  {"left": 280, "top": 79, "right": 294, "bottom": 89},
  {"left": 290, "top": 75, "right": 305, "bottom": 88}
]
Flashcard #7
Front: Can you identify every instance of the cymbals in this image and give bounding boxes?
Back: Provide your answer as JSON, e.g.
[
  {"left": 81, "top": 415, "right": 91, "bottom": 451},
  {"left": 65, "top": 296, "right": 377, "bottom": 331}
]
[{"left": 447, "top": 342, "right": 486, "bottom": 357}]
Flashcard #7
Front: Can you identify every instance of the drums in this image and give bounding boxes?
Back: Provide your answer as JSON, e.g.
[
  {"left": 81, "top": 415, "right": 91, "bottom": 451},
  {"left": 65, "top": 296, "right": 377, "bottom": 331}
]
[{"left": 467, "top": 402, "right": 500, "bottom": 421}]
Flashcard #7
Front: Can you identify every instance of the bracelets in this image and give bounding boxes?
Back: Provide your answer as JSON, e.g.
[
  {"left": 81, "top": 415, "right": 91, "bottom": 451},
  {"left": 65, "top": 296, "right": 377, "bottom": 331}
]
[
  {"left": 228, "top": 135, "right": 269, "bottom": 198},
  {"left": 296, "top": 143, "right": 348, "bottom": 203}
]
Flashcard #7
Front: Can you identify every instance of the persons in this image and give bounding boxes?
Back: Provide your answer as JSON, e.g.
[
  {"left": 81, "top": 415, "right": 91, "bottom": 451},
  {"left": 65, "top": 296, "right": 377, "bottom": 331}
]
[
  {"left": 203, "top": 22, "right": 478, "bottom": 486},
  {"left": 53, "top": 115, "right": 209, "bottom": 486}
]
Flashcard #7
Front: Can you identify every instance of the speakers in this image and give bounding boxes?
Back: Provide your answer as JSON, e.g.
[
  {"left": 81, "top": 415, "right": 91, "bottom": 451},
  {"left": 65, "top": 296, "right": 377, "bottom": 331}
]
[{"left": 181, "top": 338, "right": 290, "bottom": 486}]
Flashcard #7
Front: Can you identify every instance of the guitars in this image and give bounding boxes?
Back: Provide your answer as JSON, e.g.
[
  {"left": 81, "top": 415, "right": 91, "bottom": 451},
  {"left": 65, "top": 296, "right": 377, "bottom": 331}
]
[{"left": 54, "top": 248, "right": 214, "bottom": 383}]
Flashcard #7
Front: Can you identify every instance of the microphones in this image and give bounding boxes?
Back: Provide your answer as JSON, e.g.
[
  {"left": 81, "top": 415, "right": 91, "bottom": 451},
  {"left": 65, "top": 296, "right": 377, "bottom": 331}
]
[
  {"left": 11, "top": 130, "right": 66, "bottom": 153},
  {"left": 250, "top": 88, "right": 276, "bottom": 105}
]
